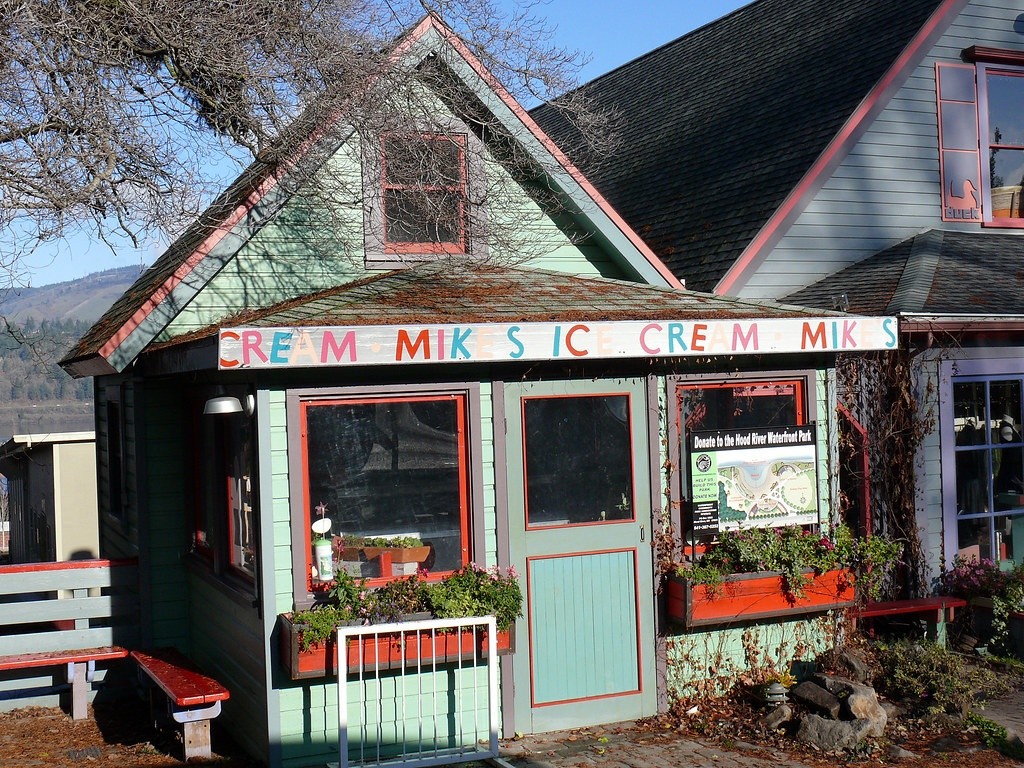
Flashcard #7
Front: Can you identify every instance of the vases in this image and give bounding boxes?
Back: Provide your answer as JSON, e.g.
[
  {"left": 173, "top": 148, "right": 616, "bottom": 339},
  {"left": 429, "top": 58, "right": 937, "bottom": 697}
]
[
  {"left": 969, "top": 596, "right": 1024, "bottom": 608},
  {"left": 667, "top": 562, "right": 859, "bottom": 630},
  {"left": 278, "top": 611, "right": 515, "bottom": 681}
]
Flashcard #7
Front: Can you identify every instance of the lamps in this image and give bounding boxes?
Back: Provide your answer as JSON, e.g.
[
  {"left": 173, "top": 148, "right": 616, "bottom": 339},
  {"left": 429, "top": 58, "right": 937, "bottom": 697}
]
[{"left": 202, "top": 385, "right": 255, "bottom": 417}]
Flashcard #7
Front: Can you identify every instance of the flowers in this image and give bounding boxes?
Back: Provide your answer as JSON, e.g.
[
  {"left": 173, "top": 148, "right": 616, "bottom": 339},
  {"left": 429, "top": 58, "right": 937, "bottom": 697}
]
[
  {"left": 289, "top": 536, "right": 523, "bottom": 653},
  {"left": 671, "top": 517, "right": 904, "bottom": 603},
  {"left": 942, "top": 552, "right": 1024, "bottom": 646}
]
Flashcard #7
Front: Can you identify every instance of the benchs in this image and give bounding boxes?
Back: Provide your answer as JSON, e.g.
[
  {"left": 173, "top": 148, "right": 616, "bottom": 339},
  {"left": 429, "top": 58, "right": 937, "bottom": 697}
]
[
  {"left": 0, "top": 559, "right": 129, "bottom": 721},
  {"left": 130, "top": 646, "right": 231, "bottom": 762},
  {"left": 845, "top": 596, "right": 967, "bottom": 641}
]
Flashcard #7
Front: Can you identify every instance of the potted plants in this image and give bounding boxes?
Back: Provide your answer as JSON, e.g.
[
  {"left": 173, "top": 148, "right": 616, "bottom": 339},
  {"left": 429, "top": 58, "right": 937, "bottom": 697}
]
[{"left": 331, "top": 534, "right": 431, "bottom": 564}]
